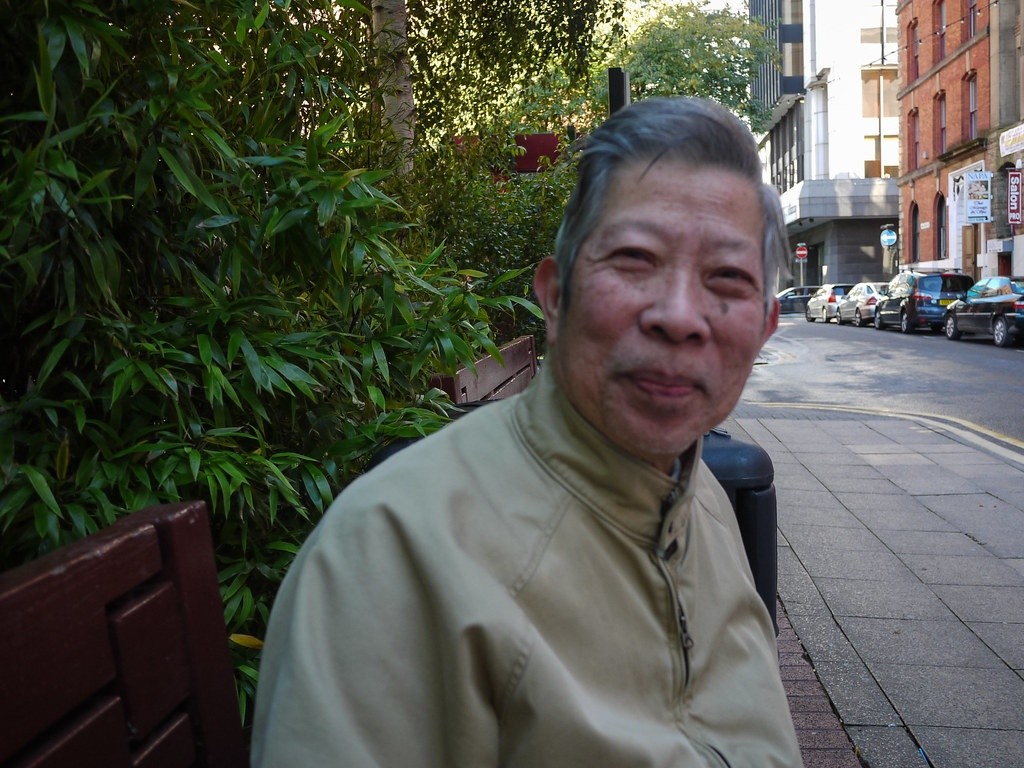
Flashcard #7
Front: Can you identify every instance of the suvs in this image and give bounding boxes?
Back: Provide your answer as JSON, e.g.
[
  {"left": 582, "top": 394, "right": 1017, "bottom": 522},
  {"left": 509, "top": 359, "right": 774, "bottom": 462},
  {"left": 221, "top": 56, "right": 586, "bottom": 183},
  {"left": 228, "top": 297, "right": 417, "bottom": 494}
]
[{"left": 873, "top": 266, "right": 975, "bottom": 334}]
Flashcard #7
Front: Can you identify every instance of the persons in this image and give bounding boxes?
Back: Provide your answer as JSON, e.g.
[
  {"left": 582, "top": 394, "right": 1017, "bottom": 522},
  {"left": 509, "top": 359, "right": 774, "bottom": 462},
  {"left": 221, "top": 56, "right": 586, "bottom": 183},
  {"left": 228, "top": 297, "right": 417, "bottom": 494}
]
[{"left": 250, "top": 95, "right": 805, "bottom": 768}]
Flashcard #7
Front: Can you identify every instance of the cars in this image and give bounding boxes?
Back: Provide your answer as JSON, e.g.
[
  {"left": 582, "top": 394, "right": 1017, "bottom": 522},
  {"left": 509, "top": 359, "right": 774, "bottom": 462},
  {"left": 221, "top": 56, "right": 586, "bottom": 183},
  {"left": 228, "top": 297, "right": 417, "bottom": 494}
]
[
  {"left": 805, "top": 282, "right": 889, "bottom": 327},
  {"left": 943, "top": 276, "right": 1024, "bottom": 348}
]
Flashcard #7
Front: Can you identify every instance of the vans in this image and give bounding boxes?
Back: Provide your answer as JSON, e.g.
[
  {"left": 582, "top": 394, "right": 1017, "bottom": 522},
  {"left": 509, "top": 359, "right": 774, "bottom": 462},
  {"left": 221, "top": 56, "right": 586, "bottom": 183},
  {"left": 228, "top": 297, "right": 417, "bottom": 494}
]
[{"left": 775, "top": 285, "right": 822, "bottom": 313}]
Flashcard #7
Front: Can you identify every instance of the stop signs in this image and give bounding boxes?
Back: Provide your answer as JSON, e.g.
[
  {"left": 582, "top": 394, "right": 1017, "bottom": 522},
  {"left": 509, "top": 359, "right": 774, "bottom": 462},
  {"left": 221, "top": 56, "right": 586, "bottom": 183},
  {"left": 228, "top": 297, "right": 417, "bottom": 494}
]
[{"left": 797, "top": 245, "right": 808, "bottom": 259}]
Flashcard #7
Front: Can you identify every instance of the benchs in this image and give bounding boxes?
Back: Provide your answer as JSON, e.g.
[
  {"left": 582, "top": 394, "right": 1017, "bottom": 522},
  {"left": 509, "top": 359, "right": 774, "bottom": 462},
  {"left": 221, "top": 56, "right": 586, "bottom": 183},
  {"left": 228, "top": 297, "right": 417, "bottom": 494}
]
[
  {"left": 453, "top": 334, "right": 541, "bottom": 418},
  {"left": 0, "top": 499, "right": 254, "bottom": 767}
]
